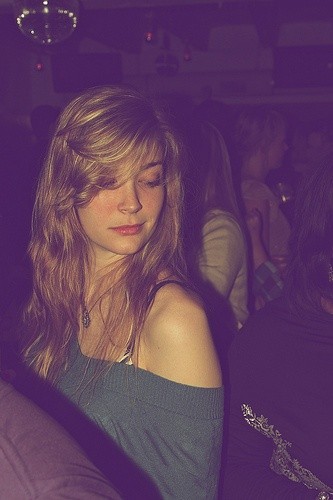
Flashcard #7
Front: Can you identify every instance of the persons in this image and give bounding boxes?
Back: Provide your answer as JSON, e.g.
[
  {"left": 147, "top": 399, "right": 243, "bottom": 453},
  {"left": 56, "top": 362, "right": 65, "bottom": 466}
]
[
  {"left": 168, "top": 111, "right": 250, "bottom": 336},
  {"left": 228, "top": 111, "right": 290, "bottom": 307},
  {"left": 16, "top": 82, "right": 225, "bottom": 499}
]
